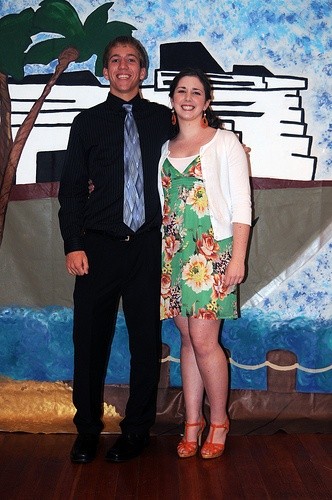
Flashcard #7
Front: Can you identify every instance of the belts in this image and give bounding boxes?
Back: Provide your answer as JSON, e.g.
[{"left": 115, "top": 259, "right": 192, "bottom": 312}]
[{"left": 86, "top": 230, "right": 150, "bottom": 248}]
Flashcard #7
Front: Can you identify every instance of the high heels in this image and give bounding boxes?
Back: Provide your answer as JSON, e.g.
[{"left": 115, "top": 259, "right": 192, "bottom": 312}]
[
  {"left": 177, "top": 416, "right": 208, "bottom": 458},
  {"left": 200, "top": 416, "right": 231, "bottom": 458}
]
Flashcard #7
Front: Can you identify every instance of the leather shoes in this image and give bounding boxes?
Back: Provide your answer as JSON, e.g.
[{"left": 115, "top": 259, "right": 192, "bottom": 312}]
[
  {"left": 71, "top": 431, "right": 102, "bottom": 464},
  {"left": 106, "top": 430, "right": 151, "bottom": 463}
]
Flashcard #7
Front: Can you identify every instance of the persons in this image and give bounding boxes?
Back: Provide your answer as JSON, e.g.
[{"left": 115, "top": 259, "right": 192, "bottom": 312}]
[
  {"left": 85, "top": 66, "right": 252, "bottom": 457},
  {"left": 58, "top": 36, "right": 252, "bottom": 465}
]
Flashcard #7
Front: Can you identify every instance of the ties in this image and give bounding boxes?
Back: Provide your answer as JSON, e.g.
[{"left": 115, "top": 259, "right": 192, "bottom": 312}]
[{"left": 120, "top": 104, "right": 146, "bottom": 232}]
